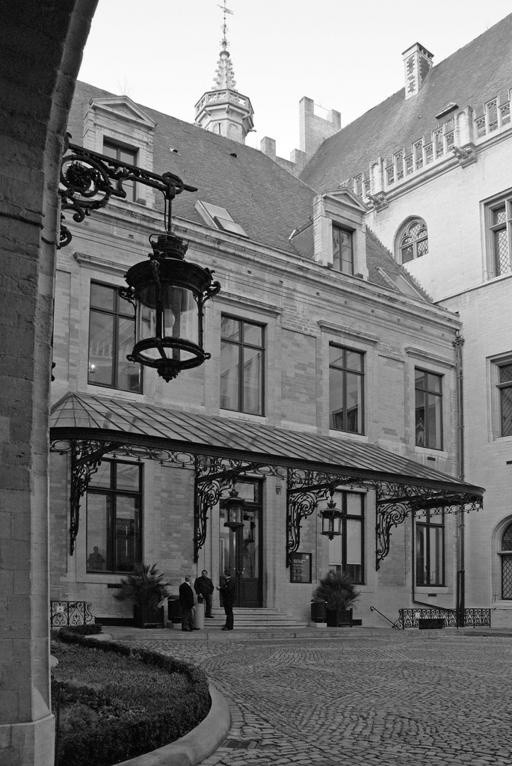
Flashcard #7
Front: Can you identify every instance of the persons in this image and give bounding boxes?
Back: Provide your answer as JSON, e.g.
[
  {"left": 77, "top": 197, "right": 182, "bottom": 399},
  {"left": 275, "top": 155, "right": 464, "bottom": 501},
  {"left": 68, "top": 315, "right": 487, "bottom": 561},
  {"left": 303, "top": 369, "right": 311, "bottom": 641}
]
[
  {"left": 87, "top": 546, "right": 105, "bottom": 570},
  {"left": 416, "top": 416, "right": 425, "bottom": 443},
  {"left": 179, "top": 569, "right": 235, "bottom": 631}
]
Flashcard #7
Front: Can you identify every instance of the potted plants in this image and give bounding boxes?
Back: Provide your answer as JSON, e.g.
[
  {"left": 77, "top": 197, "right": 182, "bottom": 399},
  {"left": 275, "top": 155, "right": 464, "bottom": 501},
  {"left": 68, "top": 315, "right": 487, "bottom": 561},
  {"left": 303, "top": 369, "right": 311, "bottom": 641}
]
[
  {"left": 116, "top": 562, "right": 172, "bottom": 624},
  {"left": 309, "top": 567, "right": 358, "bottom": 627}
]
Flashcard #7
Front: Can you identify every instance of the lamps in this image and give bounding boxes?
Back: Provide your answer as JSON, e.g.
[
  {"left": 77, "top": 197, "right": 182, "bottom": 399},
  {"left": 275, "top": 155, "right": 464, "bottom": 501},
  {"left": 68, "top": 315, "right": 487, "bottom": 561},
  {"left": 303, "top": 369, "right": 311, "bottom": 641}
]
[
  {"left": 318, "top": 489, "right": 344, "bottom": 541},
  {"left": 58, "top": 131, "right": 221, "bottom": 383},
  {"left": 224, "top": 479, "right": 246, "bottom": 534}
]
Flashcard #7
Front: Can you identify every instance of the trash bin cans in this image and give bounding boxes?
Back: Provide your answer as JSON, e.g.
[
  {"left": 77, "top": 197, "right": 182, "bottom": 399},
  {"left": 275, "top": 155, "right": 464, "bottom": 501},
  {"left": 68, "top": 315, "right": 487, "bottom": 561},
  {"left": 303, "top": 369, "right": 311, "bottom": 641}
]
[
  {"left": 311, "top": 598, "right": 328, "bottom": 623},
  {"left": 168, "top": 595, "right": 181, "bottom": 623}
]
